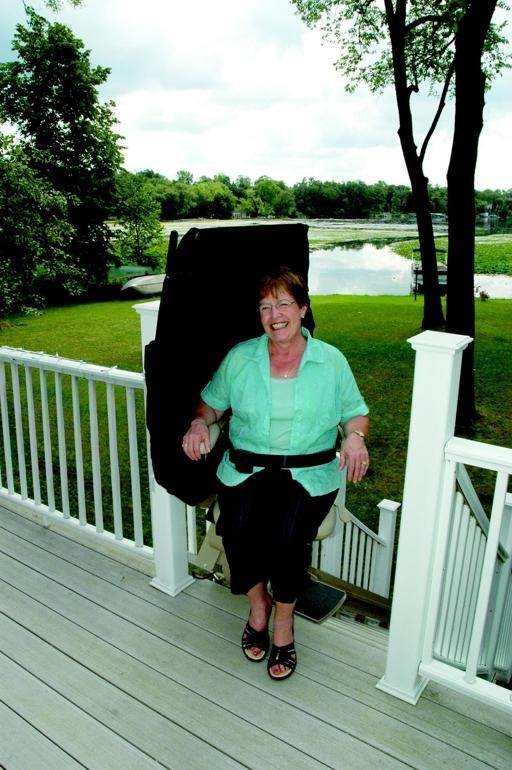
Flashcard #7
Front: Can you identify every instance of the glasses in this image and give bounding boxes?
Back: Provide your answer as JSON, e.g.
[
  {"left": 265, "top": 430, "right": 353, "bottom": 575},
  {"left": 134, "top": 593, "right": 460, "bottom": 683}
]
[{"left": 257, "top": 300, "right": 296, "bottom": 313}]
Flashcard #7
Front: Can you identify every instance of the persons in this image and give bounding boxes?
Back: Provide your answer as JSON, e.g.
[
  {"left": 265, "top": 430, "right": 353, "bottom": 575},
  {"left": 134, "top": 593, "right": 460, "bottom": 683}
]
[{"left": 176, "top": 271, "right": 371, "bottom": 681}]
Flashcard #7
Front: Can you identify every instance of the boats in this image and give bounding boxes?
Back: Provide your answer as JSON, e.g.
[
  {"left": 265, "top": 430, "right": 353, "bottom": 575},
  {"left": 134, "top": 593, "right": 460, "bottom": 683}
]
[{"left": 409, "top": 247, "right": 449, "bottom": 299}]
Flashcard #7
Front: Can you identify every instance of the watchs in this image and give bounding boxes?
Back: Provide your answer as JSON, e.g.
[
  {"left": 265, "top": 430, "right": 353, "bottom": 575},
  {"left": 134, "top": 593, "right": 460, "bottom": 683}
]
[{"left": 354, "top": 430, "right": 365, "bottom": 438}]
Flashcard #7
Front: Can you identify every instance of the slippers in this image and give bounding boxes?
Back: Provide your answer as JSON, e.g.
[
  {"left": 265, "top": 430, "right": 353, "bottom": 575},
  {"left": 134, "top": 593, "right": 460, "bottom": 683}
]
[
  {"left": 242, "top": 594, "right": 272, "bottom": 661},
  {"left": 268, "top": 626, "right": 297, "bottom": 680}
]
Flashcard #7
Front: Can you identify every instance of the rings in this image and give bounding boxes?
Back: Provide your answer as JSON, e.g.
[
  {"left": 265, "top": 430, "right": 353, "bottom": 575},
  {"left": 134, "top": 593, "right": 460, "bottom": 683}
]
[
  {"left": 361, "top": 462, "right": 367, "bottom": 465},
  {"left": 182, "top": 443, "right": 185, "bottom": 447}
]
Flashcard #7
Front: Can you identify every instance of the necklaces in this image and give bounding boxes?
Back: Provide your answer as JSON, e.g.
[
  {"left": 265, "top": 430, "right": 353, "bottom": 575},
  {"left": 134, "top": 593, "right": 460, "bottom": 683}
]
[{"left": 272, "top": 354, "right": 300, "bottom": 379}]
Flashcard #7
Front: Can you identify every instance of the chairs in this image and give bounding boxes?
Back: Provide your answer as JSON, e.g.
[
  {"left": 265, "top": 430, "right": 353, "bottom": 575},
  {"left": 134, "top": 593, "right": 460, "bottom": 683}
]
[{"left": 178, "top": 418, "right": 351, "bottom": 583}]
